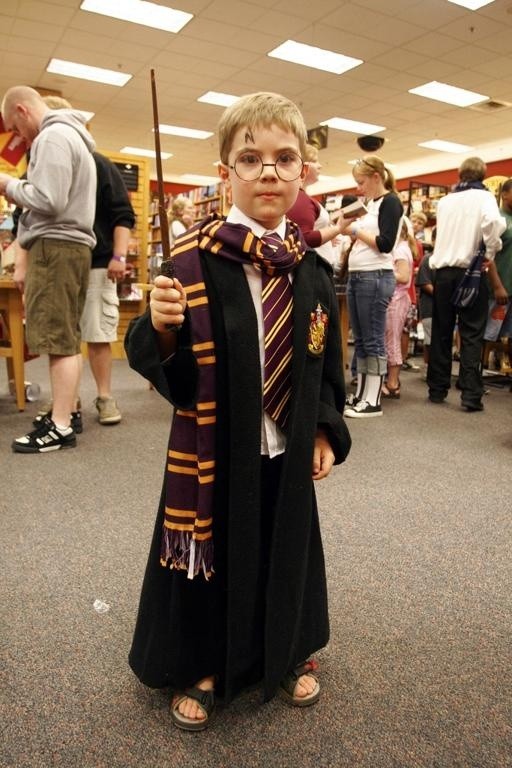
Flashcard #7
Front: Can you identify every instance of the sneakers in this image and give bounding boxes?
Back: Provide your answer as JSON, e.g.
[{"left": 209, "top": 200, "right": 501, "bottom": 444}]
[
  {"left": 402, "top": 361, "right": 420, "bottom": 372},
  {"left": 11, "top": 398, "right": 83, "bottom": 454},
  {"left": 94, "top": 394, "right": 122, "bottom": 425},
  {"left": 461, "top": 398, "right": 483, "bottom": 410},
  {"left": 343, "top": 376, "right": 400, "bottom": 418}
]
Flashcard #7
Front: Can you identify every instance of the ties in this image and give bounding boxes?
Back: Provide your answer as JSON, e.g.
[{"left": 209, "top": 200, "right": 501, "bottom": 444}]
[{"left": 261, "top": 233, "right": 296, "bottom": 435}]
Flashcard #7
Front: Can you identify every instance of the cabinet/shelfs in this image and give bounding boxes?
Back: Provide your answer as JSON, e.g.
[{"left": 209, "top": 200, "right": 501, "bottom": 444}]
[
  {"left": 80, "top": 145, "right": 151, "bottom": 361},
  {"left": 151, "top": 181, "right": 234, "bottom": 269},
  {"left": 399, "top": 180, "right": 445, "bottom": 358}
]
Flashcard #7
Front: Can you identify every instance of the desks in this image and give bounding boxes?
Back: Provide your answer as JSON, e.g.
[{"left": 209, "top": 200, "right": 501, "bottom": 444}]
[{"left": 0, "top": 274, "right": 33, "bottom": 415}]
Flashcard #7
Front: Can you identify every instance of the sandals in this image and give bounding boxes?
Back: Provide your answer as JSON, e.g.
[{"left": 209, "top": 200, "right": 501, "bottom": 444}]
[
  {"left": 169, "top": 688, "right": 217, "bottom": 732},
  {"left": 278, "top": 659, "right": 321, "bottom": 707}
]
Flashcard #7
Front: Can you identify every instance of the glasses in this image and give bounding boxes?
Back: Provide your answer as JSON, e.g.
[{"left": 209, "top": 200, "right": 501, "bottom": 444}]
[{"left": 227, "top": 150, "right": 304, "bottom": 182}]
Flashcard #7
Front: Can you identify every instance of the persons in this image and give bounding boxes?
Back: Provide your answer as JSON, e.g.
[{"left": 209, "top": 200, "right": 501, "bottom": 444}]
[
  {"left": 1, "top": 83, "right": 137, "bottom": 457},
  {"left": 146, "top": 156, "right": 512, "bottom": 419},
  {"left": 123, "top": 89, "right": 352, "bottom": 730}
]
